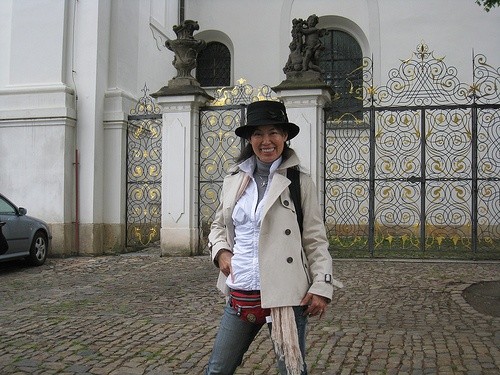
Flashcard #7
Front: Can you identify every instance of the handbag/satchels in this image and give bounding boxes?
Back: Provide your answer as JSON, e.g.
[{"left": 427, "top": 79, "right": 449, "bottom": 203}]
[{"left": 230, "top": 290, "right": 271, "bottom": 323}]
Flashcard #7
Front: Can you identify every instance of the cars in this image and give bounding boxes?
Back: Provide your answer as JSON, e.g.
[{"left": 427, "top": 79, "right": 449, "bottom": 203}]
[{"left": 0, "top": 193, "right": 52, "bottom": 275}]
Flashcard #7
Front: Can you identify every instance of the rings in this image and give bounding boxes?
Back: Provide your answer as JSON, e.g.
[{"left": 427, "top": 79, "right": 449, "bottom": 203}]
[{"left": 319, "top": 309, "right": 323, "bottom": 313}]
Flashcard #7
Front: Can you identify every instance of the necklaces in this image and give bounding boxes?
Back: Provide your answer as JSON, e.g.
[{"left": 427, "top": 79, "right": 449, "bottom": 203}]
[{"left": 259, "top": 176, "right": 268, "bottom": 188}]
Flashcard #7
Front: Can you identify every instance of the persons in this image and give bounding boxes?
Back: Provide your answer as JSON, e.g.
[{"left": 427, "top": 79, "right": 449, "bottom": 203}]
[
  {"left": 203, "top": 100, "right": 344, "bottom": 375},
  {"left": 297, "top": 14, "right": 326, "bottom": 74}
]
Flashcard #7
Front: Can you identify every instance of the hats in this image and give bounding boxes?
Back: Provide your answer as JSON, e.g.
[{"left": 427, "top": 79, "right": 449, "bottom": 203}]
[{"left": 234, "top": 101, "right": 299, "bottom": 141}]
